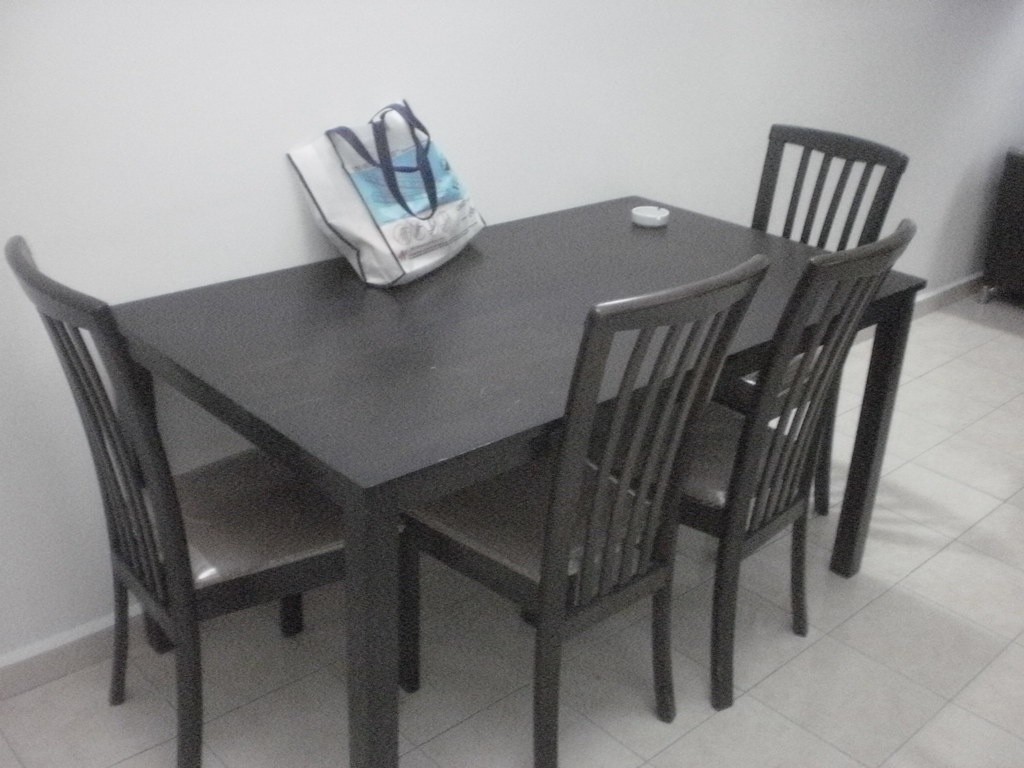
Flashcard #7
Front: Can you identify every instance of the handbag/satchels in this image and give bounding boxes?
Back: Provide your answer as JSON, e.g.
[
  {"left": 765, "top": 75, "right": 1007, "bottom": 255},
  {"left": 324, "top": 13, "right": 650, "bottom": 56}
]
[{"left": 286, "top": 101, "right": 487, "bottom": 288}]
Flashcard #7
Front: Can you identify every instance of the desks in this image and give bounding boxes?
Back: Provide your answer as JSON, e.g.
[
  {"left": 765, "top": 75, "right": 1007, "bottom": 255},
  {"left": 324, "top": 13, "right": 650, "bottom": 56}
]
[{"left": 108, "top": 196, "right": 927, "bottom": 768}]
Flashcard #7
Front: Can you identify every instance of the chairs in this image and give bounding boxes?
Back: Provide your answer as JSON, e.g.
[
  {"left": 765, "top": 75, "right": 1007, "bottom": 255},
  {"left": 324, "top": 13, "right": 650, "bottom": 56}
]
[
  {"left": 398, "top": 251, "right": 772, "bottom": 768},
  {"left": 588, "top": 218, "right": 919, "bottom": 711},
  {"left": 713, "top": 123, "right": 910, "bottom": 516},
  {"left": 5, "top": 236, "right": 407, "bottom": 768}
]
